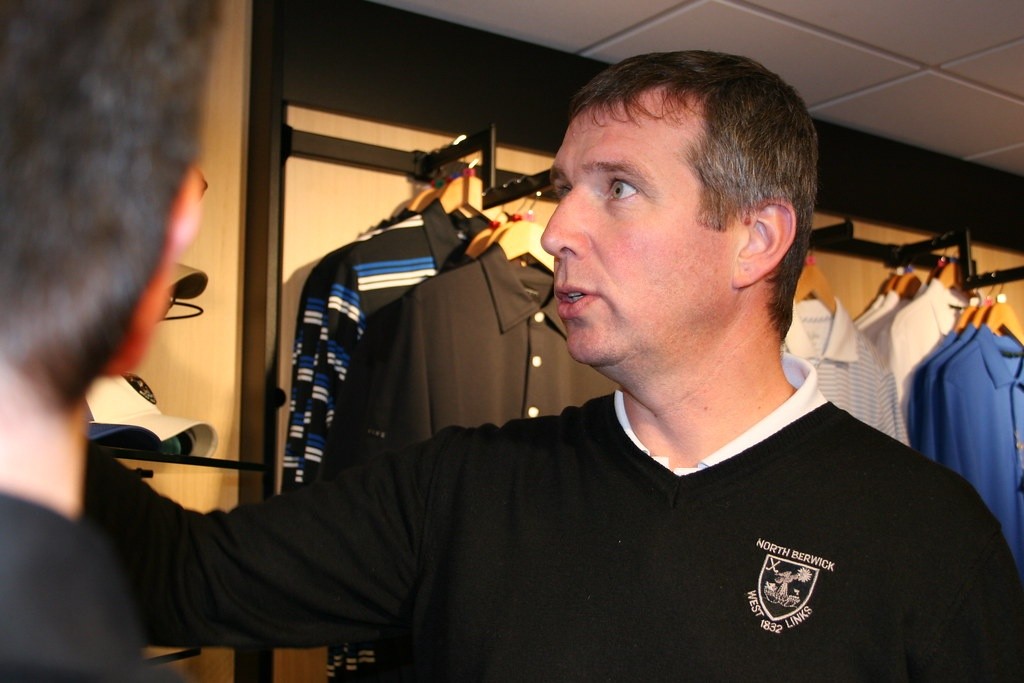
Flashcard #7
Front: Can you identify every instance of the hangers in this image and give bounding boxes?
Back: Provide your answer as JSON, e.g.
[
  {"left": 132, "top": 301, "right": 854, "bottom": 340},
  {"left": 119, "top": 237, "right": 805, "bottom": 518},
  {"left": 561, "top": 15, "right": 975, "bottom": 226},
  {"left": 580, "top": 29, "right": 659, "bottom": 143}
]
[
  {"left": 793, "top": 241, "right": 836, "bottom": 316},
  {"left": 879, "top": 232, "right": 1024, "bottom": 347},
  {"left": 386, "top": 134, "right": 555, "bottom": 274}
]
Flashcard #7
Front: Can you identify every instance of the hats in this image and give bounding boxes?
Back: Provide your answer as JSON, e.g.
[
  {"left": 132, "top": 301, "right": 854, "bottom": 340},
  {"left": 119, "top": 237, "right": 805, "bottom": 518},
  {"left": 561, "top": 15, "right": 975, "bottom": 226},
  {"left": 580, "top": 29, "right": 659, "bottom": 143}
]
[
  {"left": 88, "top": 421, "right": 163, "bottom": 455},
  {"left": 83, "top": 370, "right": 218, "bottom": 459},
  {"left": 172, "top": 262, "right": 209, "bottom": 300}
]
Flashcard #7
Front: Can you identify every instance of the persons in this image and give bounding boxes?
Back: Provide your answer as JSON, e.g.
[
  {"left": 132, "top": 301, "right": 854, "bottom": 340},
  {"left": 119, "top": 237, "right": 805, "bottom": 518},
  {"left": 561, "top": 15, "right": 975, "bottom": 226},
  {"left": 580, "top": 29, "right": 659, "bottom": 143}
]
[
  {"left": 0, "top": 0, "right": 226, "bottom": 683},
  {"left": 79, "top": 50, "right": 1024, "bottom": 683}
]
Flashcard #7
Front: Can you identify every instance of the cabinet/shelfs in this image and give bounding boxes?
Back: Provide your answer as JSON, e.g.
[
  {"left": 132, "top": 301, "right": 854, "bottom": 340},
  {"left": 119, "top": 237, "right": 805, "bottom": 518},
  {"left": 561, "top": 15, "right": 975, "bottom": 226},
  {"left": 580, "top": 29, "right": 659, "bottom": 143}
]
[{"left": 94, "top": 444, "right": 263, "bottom": 665}]
